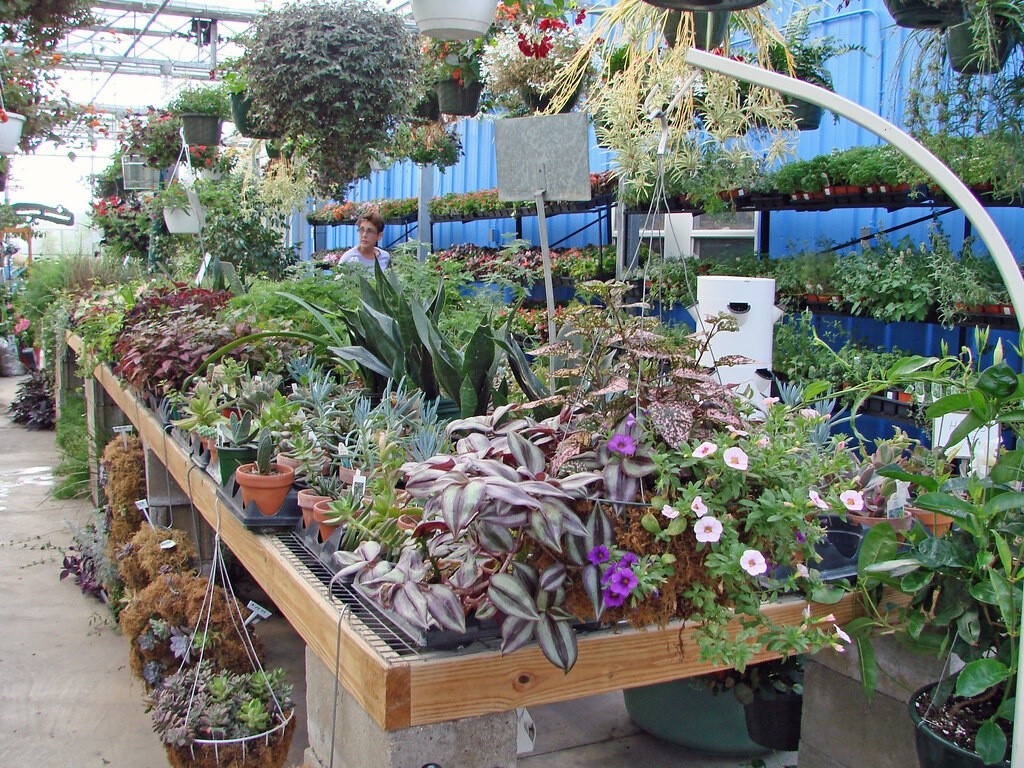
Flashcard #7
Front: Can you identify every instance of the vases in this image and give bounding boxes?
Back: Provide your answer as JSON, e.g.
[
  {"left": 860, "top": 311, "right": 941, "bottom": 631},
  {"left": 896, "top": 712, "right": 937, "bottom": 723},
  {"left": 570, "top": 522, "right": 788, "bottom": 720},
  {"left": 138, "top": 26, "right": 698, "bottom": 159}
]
[
  {"left": 433, "top": 78, "right": 484, "bottom": 116},
  {"left": 695, "top": 94, "right": 752, "bottom": 135},
  {"left": 517, "top": 79, "right": 583, "bottom": 114},
  {"left": 0, "top": 112, "right": 27, "bottom": 154},
  {"left": 161, "top": 205, "right": 208, "bottom": 233}
]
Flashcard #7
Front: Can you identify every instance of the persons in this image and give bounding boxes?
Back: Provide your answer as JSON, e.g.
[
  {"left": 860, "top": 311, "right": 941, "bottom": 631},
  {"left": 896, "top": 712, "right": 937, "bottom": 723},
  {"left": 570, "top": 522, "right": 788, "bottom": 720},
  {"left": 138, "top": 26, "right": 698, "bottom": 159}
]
[{"left": 336, "top": 212, "right": 392, "bottom": 274}]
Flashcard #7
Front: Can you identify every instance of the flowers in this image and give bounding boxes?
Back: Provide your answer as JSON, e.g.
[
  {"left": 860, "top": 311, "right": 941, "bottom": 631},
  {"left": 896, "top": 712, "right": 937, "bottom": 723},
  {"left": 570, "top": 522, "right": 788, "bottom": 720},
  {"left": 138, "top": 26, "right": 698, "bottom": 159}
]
[
  {"left": 145, "top": 177, "right": 210, "bottom": 218},
  {"left": 660, "top": 39, "right": 751, "bottom": 94},
  {"left": 474, "top": 0, "right": 606, "bottom": 113},
  {"left": 418, "top": 36, "right": 490, "bottom": 89},
  {"left": 0, "top": 43, "right": 63, "bottom": 122}
]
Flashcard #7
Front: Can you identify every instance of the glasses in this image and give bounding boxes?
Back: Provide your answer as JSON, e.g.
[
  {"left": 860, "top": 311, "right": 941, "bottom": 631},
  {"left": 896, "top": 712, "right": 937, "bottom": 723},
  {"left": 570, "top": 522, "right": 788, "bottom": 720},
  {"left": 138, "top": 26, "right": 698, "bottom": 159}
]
[{"left": 357, "top": 227, "right": 378, "bottom": 235}]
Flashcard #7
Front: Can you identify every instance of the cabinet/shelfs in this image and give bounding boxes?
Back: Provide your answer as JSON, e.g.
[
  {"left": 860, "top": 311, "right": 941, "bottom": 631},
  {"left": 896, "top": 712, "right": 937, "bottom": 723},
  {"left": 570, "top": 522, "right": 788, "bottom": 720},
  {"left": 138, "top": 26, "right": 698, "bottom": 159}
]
[
  {"left": 306, "top": 200, "right": 615, "bottom": 279},
  {"left": 622, "top": 194, "right": 1024, "bottom": 477}
]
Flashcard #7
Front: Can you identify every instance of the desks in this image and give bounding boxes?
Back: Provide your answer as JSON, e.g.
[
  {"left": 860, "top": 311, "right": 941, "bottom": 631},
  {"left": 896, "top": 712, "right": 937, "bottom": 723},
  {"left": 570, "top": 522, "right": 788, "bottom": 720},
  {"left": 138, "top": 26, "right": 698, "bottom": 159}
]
[{"left": 67, "top": 331, "right": 940, "bottom": 768}]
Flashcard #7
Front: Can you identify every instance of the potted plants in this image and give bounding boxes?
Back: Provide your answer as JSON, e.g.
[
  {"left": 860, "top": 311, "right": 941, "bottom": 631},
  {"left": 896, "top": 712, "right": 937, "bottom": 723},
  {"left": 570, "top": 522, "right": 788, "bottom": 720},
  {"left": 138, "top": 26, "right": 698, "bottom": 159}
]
[{"left": 76, "top": 0, "right": 1024, "bottom": 768}]
